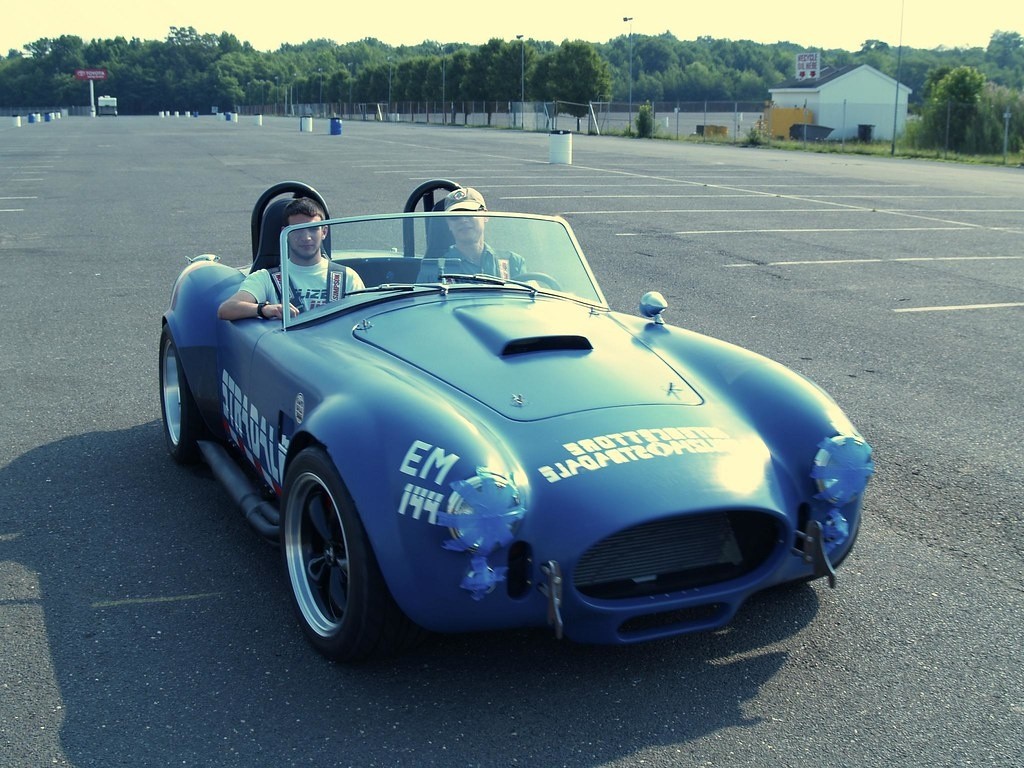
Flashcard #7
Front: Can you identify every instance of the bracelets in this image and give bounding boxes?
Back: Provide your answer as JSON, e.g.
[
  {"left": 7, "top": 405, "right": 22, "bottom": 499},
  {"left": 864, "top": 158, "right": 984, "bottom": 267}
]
[{"left": 257, "top": 300, "right": 271, "bottom": 320}]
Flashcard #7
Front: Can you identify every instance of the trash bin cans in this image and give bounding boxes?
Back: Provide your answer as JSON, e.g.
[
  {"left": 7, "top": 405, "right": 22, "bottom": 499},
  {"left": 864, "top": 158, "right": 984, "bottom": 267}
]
[
  {"left": 253, "top": 113, "right": 263, "bottom": 127},
  {"left": 166, "top": 111, "right": 170, "bottom": 116},
  {"left": 225, "top": 111, "right": 231, "bottom": 121},
  {"left": 11, "top": 114, "right": 22, "bottom": 127},
  {"left": 44, "top": 112, "right": 51, "bottom": 121},
  {"left": 159, "top": 111, "right": 165, "bottom": 118},
  {"left": 329, "top": 117, "right": 342, "bottom": 135},
  {"left": 299, "top": 115, "right": 313, "bottom": 132},
  {"left": 55, "top": 111, "right": 61, "bottom": 119},
  {"left": 216, "top": 112, "right": 224, "bottom": 121},
  {"left": 548, "top": 129, "right": 573, "bottom": 165},
  {"left": 49, "top": 111, "right": 55, "bottom": 120},
  {"left": 185, "top": 111, "right": 190, "bottom": 118},
  {"left": 230, "top": 112, "right": 238, "bottom": 122},
  {"left": 174, "top": 111, "right": 179, "bottom": 118},
  {"left": 192, "top": 111, "right": 198, "bottom": 117},
  {"left": 28, "top": 112, "right": 41, "bottom": 123}
]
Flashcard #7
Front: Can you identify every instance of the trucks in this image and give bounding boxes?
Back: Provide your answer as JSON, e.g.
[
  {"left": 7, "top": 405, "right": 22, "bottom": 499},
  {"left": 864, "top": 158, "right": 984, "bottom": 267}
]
[{"left": 98, "top": 96, "right": 118, "bottom": 117}]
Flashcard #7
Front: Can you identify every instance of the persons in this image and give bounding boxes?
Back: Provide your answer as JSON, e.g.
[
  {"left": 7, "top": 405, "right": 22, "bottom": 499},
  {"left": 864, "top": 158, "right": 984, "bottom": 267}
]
[
  {"left": 416, "top": 188, "right": 537, "bottom": 286},
  {"left": 217, "top": 196, "right": 366, "bottom": 320}
]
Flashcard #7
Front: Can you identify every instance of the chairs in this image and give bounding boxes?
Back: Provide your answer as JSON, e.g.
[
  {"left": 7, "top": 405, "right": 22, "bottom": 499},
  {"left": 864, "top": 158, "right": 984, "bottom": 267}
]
[
  {"left": 424, "top": 198, "right": 455, "bottom": 258},
  {"left": 249, "top": 198, "right": 294, "bottom": 272}
]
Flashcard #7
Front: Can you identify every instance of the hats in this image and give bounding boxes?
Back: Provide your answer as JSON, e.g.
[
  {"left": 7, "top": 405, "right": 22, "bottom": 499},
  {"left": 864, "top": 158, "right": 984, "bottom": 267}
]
[{"left": 444, "top": 187, "right": 486, "bottom": 211}]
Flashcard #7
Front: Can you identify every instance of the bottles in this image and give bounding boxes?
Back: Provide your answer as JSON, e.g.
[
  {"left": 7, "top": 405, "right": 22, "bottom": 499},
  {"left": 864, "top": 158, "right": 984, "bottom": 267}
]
[{"left": 439, "top": 266, "right": 446, "bottom": 284}]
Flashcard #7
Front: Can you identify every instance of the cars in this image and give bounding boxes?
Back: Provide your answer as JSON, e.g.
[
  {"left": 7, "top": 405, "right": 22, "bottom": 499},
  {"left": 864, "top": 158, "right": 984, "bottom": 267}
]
[{"left": 156, "top": 179, "right": 875, "bottom": 667}]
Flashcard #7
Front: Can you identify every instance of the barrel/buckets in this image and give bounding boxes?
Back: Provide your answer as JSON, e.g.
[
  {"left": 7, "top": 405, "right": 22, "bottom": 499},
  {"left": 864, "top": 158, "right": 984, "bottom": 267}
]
[
  {"left": 159, "top": 111, "right": 164, "bottom": 118},
  {"left": 174, "top": 111, "right": 179, "bottom": 119},
  {"left": 217, "top": 112, "right": 238, "bottom": 123},
  {"left": 185, "top": 111, "right": 191, "bottom": 118},
  {"left": 254, "top": 114, "right": 263, "bottom": 126},
  {"left": 300, "top": 115, "right": 313, "bottom": 132},
  {"left": 44, "top": 111, "right": 61, "bottom": 122},
  {"left": 330, "top": 118, "right": 342, "bottom": 135},
  {"left": 550, "top": 130, "right": 573, "bottom": 164},
  {"left": 12, "top": 115, "right": 21, "bottom": 127},
  {"left": 193, "top": 110, "right": 198, "bottom": 117},
  {"left": 166, "top": 111, "right": 170, "bottom": 117},
  {"left": 28, "top": 112, "right": 41, "bottom": 123}
]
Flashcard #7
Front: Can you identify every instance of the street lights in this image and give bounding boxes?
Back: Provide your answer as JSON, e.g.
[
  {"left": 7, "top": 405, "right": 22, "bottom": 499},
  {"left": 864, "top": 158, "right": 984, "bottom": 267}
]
[
  {"left": 387, "top": 57, "right": 391, "bottom": 122},
  {"left": 439, "top": 46, "right": 448, "bottom": 125},
  {"left": 260, "top": 79, "right": 264, "bottom": 105},
  {"left": 623, "top": 17, "right": 634, "bottom": 135},
  {"left": 348, "top": 63, "right": 352, "bottom": 120},
  {"left": 294, "top": 74, "right": 299, "bottom": 105},
  {"left": 516, "top": 34, "right": 527, "bottom": 131},
  {"left": 318, "top": 68, "right": 322, "bottom": 102},
  {"left": 275, "top": 76, "right": 279, "bottom": 104}
]
